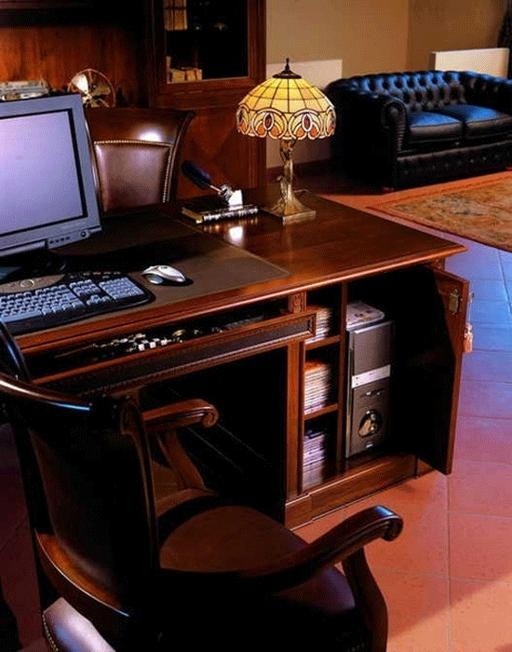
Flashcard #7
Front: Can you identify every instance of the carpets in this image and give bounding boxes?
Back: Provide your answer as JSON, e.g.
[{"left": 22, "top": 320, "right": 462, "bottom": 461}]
[{"left": 362, "top": 168, "right": 512, "bottom": 252}]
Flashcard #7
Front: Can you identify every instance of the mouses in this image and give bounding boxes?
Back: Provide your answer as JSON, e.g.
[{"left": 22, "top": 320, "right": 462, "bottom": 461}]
[{"left": 140, "top": 263, "right": 187, "bottom": 285}]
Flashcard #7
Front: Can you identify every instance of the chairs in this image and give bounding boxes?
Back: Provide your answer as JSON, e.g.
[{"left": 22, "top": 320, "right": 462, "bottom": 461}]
[
  {"left": 82, "top": 102, "right": 197, "bottom": 213},
  {"left": 0, "top": 368, "right": 405, "bottom": 651}
]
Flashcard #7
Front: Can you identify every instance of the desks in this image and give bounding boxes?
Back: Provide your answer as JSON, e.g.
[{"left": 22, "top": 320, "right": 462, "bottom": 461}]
[{"left": 0, "top": 182, "right": 473, "bottom": 528}]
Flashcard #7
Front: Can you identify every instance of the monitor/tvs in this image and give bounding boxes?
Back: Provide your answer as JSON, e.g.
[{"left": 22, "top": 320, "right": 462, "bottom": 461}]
[{"left": 0, "top": 93, "right": 104, "bottom": 294}]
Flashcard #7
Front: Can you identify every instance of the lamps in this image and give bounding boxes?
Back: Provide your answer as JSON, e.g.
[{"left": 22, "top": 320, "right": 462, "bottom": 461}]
[{"left": 234, "top": 56, "right": 338, "bottom": 227}]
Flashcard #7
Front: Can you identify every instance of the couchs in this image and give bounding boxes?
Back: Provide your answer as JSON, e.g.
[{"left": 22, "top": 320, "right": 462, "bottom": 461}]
[{"left": 327, "top": 68, "right": 511, "bottom": 193}]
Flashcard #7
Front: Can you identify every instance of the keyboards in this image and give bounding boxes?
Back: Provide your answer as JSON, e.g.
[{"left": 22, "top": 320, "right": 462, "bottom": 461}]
[{"left": 0, "top": 274, "right": 155, "bottom": 336}]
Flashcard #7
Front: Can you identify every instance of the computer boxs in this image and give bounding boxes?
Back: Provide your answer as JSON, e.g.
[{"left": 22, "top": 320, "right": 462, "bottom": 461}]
[{"left": 346, "top": 318, "right": 399, "bottom": 459}]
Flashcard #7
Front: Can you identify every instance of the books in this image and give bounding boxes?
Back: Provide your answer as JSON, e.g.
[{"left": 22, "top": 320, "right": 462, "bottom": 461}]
[
  {"left": 303, "top": 428, "right": 325, "bottom": 472},
  {"left": 346, "top": 303, "right": 384, "bottom": 329},
  {"left": 304, "top": 306, "right": 331, "bottom": 345},
  {"left": 304, "top": 361, "right": 330, "bottom": 415}
]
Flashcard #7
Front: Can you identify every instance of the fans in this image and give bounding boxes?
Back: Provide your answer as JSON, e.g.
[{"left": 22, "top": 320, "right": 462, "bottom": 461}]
[{"left": 65, "top": 63, "right": 119, "bottom": 110}]
